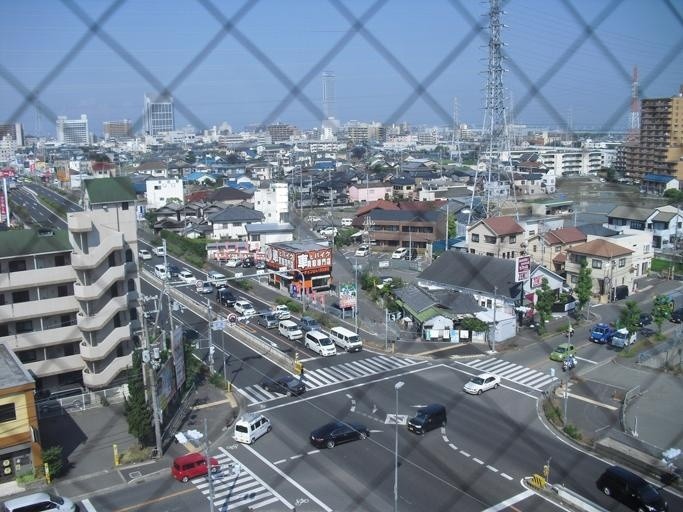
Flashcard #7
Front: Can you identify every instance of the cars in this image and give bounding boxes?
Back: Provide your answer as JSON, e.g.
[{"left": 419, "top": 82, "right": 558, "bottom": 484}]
[
  {"left": 670, "top": 308, "right": 683, "bottom": 323},
  {"left": 139, "top": 240, "right": 267, "bottom": 320},
  {"left": 261, "top": 369, "right": 306, "bottom": 397},
  {"left": 563, "top": 283, "right": 572, "bottom": 293},
  {"left": 9, "top": 174, "right": 35, "bottom": 184},
  {"left": 463, "top": 373, "right": 501, "bottom": 396},
  {"left": 310, "top": 420, "right": 370, "bottom": 449},
  {"left": 306, "top": 214, "right": 419, "bottom": 260},
  {"left": 637, "top": 313, "right": 653, "bottom": 326}
]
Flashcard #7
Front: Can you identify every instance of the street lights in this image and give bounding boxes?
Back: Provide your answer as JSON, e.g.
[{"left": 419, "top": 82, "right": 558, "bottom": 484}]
[
  {"left": 561, "top": 321, "right": 574, "bottom": 431},
  {"left": 394, "top": 380, "right": 405, "bottom": 502}
]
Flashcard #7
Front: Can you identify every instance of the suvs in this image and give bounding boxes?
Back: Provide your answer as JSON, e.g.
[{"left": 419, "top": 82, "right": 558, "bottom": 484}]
[{"left": 550, "top": 343, "right": 575, "bottom": 362}]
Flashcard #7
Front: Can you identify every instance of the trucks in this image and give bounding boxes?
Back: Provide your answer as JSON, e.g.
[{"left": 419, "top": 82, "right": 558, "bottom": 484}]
[{"left": 588, "top": 322, "right": 619, "bottom": 344}]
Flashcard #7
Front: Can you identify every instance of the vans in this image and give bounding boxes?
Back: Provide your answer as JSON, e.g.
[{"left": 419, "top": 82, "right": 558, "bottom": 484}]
[
  {"left": 233, "top": 412, "right": 273, "bottom": 445},
  {"left": 33, "top": 383, "right": 86, "bottom": 415},
  {"left": 257, "top": 303, "right": 363, "bottom": 358},
  {"left": 408, "top": 403, "right": 447, "bottom": 436},
  {"left": 611, "top": 326, "right": 637, "bottom": 348},
  {"left": 4, "top": 493, "right": 77, "bottom": 512},
  {"left": 599, "top": 466, "right": 670, "bottom": 512},
  {"left": 171, "top": 453, "right": 220, "bottom": 484}
]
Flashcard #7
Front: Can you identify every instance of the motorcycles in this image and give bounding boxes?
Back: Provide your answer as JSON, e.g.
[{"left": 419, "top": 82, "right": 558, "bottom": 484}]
[{"left": 562, "top": 358, "right": 578, "bottom": 371}]
